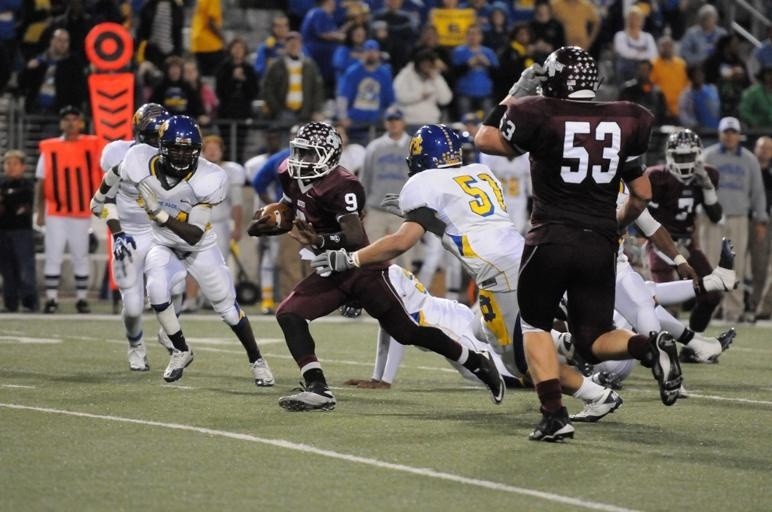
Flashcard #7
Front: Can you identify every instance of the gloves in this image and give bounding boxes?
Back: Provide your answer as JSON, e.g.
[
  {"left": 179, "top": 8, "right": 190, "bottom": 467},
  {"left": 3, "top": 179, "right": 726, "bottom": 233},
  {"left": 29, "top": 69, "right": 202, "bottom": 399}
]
[
  {"left": 114, "top": 231, "right": 137, "bottom": 259},
  {"left": 509, "top": 63, "right": 548, "bottom": 96},
  {"left": 137, "top": 182, "right": 169, "bottom": 224},
  {"left": 380, "top": 193, "right": 406, "bottom": 219},
  {"left": 695, "top": 164, "right": 715, "bottom": 192},
  {"left": 310, "top": 248, "right": 360, "bottom": 276}
]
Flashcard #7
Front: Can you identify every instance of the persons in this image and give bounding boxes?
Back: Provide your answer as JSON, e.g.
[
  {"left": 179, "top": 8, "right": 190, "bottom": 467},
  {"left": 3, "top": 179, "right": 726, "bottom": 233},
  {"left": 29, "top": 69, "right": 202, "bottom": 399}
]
[
  {"left": 336, "top": 106, "right": 737, "bottom": 389},
  {"left": 247, "top": 124, "right": 506, "bottom": 412},
  {"left": 91, "top": 115, "right": 274, "bottom": 387},
  {"left": 312, "top": 123, "right": 623, "bottom": 421},
  {"left": 476, "top": 45, "right": 683, "bottom": 444},
  {"left": 0, "top": 1, "right": 566, "bottom": 314},
  {"left": 100, "top": 104, "right": 185, "bottom": 371},
  {"left": 593, "top": 1, "right": 772, "bottom": 326}
]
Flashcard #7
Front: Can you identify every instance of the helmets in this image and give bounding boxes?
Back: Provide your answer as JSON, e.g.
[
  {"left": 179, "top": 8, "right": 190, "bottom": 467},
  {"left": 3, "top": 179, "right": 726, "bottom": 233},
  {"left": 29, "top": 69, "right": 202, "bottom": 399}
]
[
  {"left": 132, "top": 102, "right": 169, "bottom": 142},
  {"left": 288, "top": 121, "right": 343, "bottom": 180},
  {"left": 160, "top": 114, "right": 203, "bottom": 175},
  {"left": 666, "top": 128, "right": 704, "bottom": 186},
  {"left": 541, "top": 45, "right": 599, "bottom": 99},
  {"left": 405, "top": 124, "right": 464, "bottom": 176}
]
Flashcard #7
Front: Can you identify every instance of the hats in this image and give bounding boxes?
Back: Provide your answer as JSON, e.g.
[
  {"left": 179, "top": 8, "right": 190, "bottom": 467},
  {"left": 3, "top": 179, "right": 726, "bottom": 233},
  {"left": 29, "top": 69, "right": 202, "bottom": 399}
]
[
  {"left": 384, "top": 106, "right": 403, "bottom": 120},
  {"left": 719, "top": 116, "right": 741, "bottom": 133}
]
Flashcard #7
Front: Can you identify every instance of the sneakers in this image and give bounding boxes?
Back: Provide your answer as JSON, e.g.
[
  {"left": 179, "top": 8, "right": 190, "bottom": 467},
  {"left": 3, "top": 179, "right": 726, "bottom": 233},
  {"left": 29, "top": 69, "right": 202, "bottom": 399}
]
[
  {"left": 529, "top": 406, "right": 574, "bottom": 442},
  {"left": 713, "top": 237, "right": 740, "bottom": 292},
  {"left": 279, "top": 386, "right": 336, "bottom": 412},
  {"left": 470, "top": 350, "right": 506, "bottom": 405},
  {"left": 640, "top": 330, "right": 683, "bottom": 406},
  {"left": 253, "top": 358, "right": 275, "bottom": 386},
  {"left": 128, "top": 330, "right": 193, "bottom": 382},
  {"left": 678, "top": 383, "right": 688, "bottom": 398},
  {"left": 556, "top": 332, "right": 593, "bottom": 377},
  {"left": 77, "top": 299, "right": 90, "bottom": 312},
  {"left": 43, "top": 299, "right": 57, "bottom": 312},
  {"left": 695, "top": 327, "right": 736, "bottom": 364},
  {"left": 569, "top": 388, "right": 623, "bottom": 423},
  {"left": 592, "top": 370, "right": 623, "bottom": 391},
  {"left": 679, "top": 347, "right": 720, "bottom": 365}
]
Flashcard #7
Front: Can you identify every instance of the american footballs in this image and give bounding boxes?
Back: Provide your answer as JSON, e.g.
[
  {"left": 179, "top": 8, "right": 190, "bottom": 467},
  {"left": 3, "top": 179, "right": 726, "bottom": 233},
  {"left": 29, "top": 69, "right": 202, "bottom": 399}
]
[{"left": 259, "top": 203, "right": 291, "bottom": 230}]
